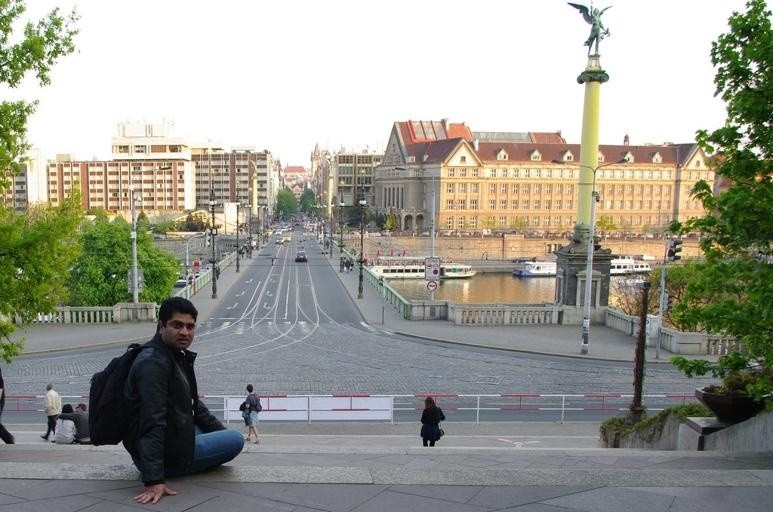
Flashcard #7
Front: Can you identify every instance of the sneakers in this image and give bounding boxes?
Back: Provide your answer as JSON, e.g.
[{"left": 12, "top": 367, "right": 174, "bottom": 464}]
[
  {"left": 245, "top": 438, "right": 251, "bottom": 441},
  {"left": 254, "top": 440, "right": 259, "bottom": 444},
  {"left": 40, "top": 436, "right": 47, "bottom": 440}
]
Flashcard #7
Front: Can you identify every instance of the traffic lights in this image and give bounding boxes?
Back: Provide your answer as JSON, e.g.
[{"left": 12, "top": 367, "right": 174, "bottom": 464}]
[{"left": 673, "top": 239, "right": 683, "bottom": 263}]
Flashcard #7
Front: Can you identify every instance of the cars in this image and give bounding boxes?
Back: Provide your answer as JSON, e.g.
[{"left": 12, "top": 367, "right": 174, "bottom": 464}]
[{"left": 295, "top": 251, "right": 307, "bottom": 262}]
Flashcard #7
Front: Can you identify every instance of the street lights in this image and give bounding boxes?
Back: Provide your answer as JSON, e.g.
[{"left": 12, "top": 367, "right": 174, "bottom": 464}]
[
  {"left": 339, "top": 199, "right": 345, "bottom": 257},
  {"left": 357, "top": 182, "right": 367, "bottom": 300},
  {"left": 208, "top": 188, "right": 218, "bottom": 299},
  {"left": 236, "top": 201, "right": 241, "bottom": 273}
]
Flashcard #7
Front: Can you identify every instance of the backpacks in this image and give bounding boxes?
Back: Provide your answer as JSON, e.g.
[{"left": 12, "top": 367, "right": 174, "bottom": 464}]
[{"left": 87, "top": 342, "right": 172, "bottom": 446}]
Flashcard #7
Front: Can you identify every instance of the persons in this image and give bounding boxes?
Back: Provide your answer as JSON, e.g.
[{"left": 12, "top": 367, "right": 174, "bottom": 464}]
[
  {"left": 52, "top": 404, "right": 76, "bottom": 444},
  {"left": 419, "top": 397, "right": 445, "bottom": 446},
  {"left": 243, "top": 384, "right": 260, "bottom": 444},
  {"left": 0, "top": 368, "right": 15, "bottom": 445},
  {"left": 39, "top": 384, "right": 61, "bottom": 442},
  {"left": 584, "top": 6, "right": 611, "bottom": 56},
  {"left": 120, "top": 296, "right": 244, "bottom": 504},
  {"left": 54, "top": 403, "right": 93, "bottom": 445}
]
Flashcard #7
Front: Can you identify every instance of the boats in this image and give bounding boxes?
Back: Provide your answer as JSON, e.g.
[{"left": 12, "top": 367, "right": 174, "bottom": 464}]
[
  {"left": 512, "top": 256, "right": 652, "bottom": 279},
  {"left": 370, "top": 263, "right": 477, "bottom": 279}
]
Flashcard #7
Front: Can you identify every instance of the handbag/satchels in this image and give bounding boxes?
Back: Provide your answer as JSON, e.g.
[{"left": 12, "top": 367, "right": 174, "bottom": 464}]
[
  {"left": 439, "top": 429, "right": 444, "bottom": 435},
  {"left": 255, "top": 403, "right": 262, "bottom": 412}
]
